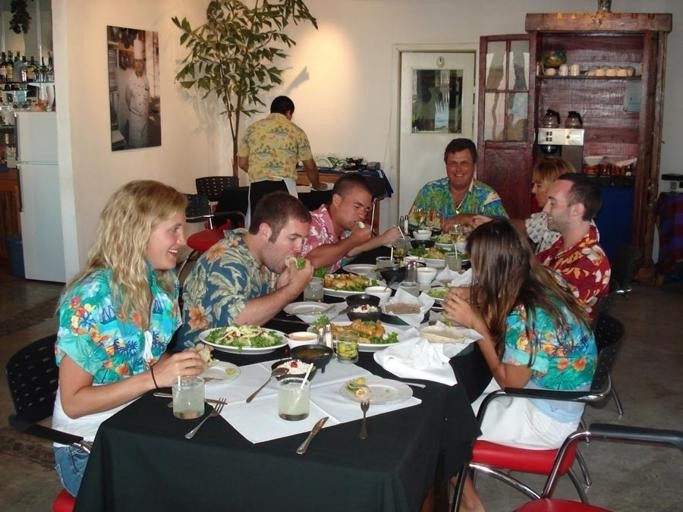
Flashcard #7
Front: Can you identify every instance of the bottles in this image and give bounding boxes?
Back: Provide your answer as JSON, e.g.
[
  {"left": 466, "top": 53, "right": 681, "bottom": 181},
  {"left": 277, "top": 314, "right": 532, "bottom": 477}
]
[
  {"left": 585, "top": 162, "right": 634, "bottom": 186},
  {"left": 0, "top": 48, "right": 54, "bottom": 127}
]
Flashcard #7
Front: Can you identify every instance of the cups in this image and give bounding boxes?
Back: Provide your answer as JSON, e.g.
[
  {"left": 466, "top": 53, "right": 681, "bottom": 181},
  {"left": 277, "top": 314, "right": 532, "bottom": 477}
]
[
  {"left": 276, "top": 375, "right": 310, "bottom": 422},
  {"left": 336, "top": 329, "right": 360, "bottom": 365},
  {"left": 542, "top": 63, "right": 635, "bottom": 78},
  {"left": 168, "top": 372, "right": 206, "bottom": 420}
]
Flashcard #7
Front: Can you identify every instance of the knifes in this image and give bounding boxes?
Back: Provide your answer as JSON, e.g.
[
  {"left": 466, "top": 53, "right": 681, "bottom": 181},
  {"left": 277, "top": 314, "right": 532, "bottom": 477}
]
[{"left": 296, "top": 416, "right": 330, "bottom": 455}]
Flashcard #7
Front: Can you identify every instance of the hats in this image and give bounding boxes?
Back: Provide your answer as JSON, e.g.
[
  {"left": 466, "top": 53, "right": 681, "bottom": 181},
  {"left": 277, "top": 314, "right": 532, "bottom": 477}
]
[{"left": 132, "top": 39, "right": 146, "bottom": 60}]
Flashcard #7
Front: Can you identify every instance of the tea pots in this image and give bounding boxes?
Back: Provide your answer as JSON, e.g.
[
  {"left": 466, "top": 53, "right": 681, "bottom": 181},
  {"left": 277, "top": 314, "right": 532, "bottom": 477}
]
[{"left": 541, "top": 107, "right": 586, "bottom": 129}]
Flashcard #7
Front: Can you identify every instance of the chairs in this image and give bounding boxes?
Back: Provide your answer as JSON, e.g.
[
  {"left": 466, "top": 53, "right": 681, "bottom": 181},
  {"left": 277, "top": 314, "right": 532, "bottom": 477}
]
[
  {"left": 176, "top": 212, "right": 245, "bottom": 282},
  {"left": 9, "top": 331, "right": 93, "bottom": 512},
  {"left": 519, "top": 424, "right": 683, "bottom": 512},
  {"left": 452, "top": 311, "right": 624, "bottom": 512}
]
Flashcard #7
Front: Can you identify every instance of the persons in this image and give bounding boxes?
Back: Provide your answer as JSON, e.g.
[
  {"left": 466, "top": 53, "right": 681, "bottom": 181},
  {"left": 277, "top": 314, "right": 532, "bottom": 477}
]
[
  {"left": 173, "top": 190, "right": 314, "bottom": 353},
  {"left": 407, "top": 138, "right": 510, "bottom": 230},
  {"left": 125, "top": 59, "right": 151, "bottom": 148},
  {"left": 48, "top": 179, "right": 204, "bottom": 499},
  {"left": 341, "top": 226, "right": 402, "bottom": 265},
  {"left": 511, "top": 155, "right": 601, "bottom": 253},
  {"left": 296, "top": 172, "right": 373, "bottom": 276},
  {"left": 235, "top": 96, "right": 327, "bottom": 209},
  {"left": 440, "top": 216, "right": 599, "bottom": 512},
  {"left": 472, "top": 172, "right": 612, "bottom": 327}
]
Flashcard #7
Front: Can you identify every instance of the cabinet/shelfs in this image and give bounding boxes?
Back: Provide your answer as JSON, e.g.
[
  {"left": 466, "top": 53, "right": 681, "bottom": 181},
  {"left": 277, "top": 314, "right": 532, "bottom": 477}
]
[
  {"left": 1, "top": 169, "right": 19, "bottom": 266},
  {"left": 475, "top": 13, "right": 671, "bottom": 289},
  {"left": 295, "top": 166, "right": 382, "bottom": 233}
]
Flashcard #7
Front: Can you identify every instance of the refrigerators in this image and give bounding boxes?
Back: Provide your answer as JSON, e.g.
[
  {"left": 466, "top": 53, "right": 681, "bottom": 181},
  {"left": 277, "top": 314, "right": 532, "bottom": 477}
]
[{"left": 10, "top": 112, "right": 64, "bottom": 283}]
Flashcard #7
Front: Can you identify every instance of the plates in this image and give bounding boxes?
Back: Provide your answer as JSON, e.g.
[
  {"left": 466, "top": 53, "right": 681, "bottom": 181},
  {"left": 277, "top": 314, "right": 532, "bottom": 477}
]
[
  {"left": 313, "top": 149, "right": 346, "bottom": 168},
  {"left": 198, "top": 324, "right": 289, "bottom": 355},
  {"left": 282, "top": 207, "right": 478, "bottom": 318},
  {"left": 308, "top": 320, "right": 406, "bottom": 352},
  {"left": 193, "top": 359, "right": 242, "bottom": 388},
  {"left": 340, "top": 376, "right": 414, "bottom": 407}
]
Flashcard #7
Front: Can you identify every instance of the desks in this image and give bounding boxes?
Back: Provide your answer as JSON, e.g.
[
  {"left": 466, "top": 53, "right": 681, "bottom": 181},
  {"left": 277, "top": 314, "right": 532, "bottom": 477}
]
[{"left": 74, "top": 239, "right": 533, "bottom": 512}]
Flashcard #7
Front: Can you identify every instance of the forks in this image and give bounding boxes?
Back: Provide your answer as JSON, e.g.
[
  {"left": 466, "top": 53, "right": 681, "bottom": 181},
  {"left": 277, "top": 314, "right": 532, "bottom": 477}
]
[
  {"left": 184, "top": 396, "right": 226, "bottom": 438},
  {"left": 359, "top": 402, "right": 372, "bottom": 440}
]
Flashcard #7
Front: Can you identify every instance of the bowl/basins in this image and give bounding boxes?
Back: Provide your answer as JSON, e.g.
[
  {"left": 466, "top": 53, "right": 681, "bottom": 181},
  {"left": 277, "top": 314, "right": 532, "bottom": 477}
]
[
  {"left": 286, "top": 331, "right": 319, "bottom": 349},
  {"left": 288, "top": 344, "right": 334, "bottom": 375},
  {"left": 270, "top": 360, "right": 317, "bottom": 384}
]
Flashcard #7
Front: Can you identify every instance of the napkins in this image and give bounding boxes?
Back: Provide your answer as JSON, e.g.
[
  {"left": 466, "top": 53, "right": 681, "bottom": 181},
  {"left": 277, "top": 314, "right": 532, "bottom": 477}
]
[{"left": 372, "top": 337, "right": 457, "bottom": 386}]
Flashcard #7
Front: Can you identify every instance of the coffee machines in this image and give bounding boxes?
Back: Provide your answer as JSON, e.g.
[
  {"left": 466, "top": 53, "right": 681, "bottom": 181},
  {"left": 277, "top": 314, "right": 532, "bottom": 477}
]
[{"left": 537, "top": 129, "right": 584, "bottom": 173}]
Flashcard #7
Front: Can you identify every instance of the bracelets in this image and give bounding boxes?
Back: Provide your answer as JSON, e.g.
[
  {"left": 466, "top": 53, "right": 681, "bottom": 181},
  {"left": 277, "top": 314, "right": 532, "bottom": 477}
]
[{"left": 148, "top": 367, "right": 160, "bottom": 392}]
